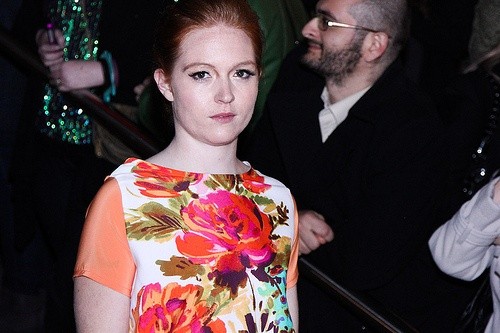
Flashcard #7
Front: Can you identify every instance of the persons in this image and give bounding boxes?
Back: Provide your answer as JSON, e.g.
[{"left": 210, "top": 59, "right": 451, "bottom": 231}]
[
  {"left": 0, "top": 0, "right": 175, "bottom": 333},
  {"left": 258, "top": 0, "right": 500, "bottom": 333},
  {"left": 70, "top": 0, "right": 304, "bottom": 333}
]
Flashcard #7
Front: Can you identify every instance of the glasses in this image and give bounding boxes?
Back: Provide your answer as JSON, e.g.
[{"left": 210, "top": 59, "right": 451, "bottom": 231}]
[{"left": 312, "top": 12, "right": 392, "bottom": 39}]
